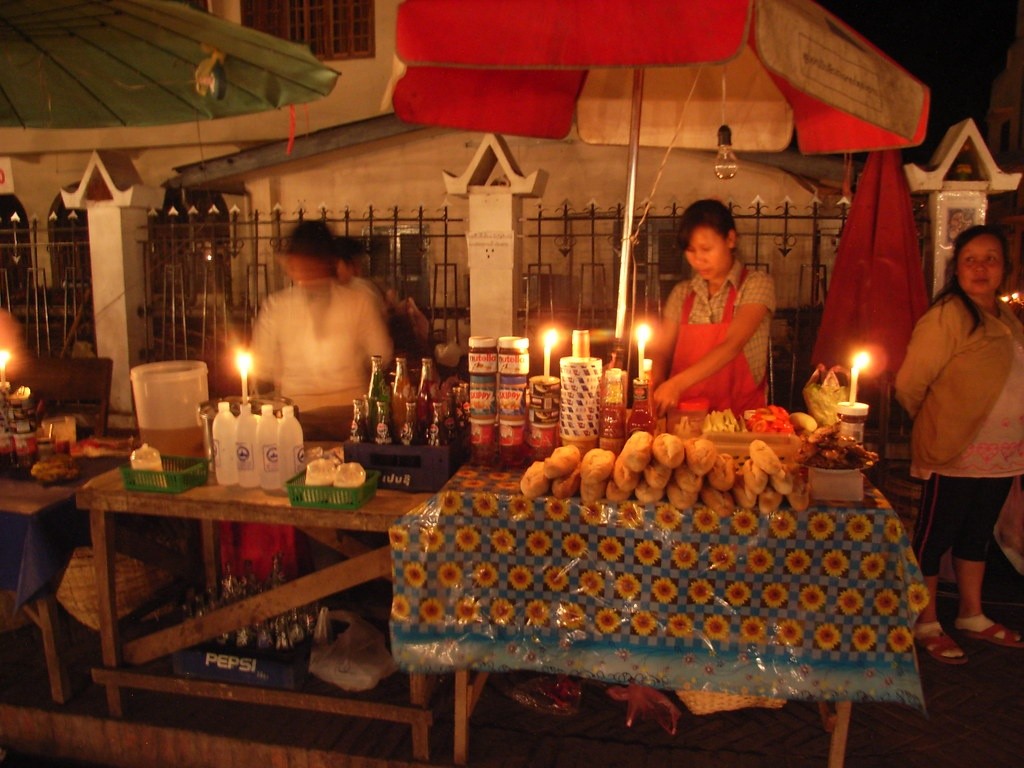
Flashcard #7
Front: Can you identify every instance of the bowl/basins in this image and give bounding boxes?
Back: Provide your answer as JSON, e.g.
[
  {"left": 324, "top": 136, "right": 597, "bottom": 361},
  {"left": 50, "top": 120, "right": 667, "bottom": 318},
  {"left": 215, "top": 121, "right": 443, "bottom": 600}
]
[{"left": 797, "top": 460, "right": 877, "bottom": 490}]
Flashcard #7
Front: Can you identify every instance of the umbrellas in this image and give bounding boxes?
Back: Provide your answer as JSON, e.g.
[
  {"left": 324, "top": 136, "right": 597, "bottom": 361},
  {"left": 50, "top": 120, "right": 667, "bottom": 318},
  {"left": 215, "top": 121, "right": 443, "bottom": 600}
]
[
  {"left": 376, "top": 0, "right": 930, "bottom": 372},
  {"left": 0, "top": 0, "right": 342, "bottom": 129},
  {"left": 810, "top": 146, "right": 929, "bottom": 435}
]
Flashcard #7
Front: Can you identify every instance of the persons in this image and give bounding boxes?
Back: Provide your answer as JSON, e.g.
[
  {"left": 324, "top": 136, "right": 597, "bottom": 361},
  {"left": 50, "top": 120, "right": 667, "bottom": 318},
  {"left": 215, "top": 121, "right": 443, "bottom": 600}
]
[
  {"left": 892, "top": 224, "right": 1024, "bottom": 663},
  {"left": 649, "top": 200, "right": 786, "bottom": 715},
  {"left": 247, "top": 219, "right": 437, "bottom": 446}
]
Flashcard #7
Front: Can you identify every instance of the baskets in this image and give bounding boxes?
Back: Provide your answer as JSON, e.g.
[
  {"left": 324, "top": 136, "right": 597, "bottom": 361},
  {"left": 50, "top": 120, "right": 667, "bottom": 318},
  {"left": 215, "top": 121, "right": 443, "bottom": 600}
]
[
  {"left": 284, "top": 466, "right": 380, "bottom": 509},
  {"left": 120, "top": 455, "right": 209, "bottom": 493}
]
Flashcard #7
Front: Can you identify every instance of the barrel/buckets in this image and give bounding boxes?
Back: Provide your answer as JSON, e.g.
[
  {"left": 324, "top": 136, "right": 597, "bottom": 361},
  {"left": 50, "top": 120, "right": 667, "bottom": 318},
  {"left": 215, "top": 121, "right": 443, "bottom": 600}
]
[{"left": 130, "top": 359, "right": 211, "bottom": 463}]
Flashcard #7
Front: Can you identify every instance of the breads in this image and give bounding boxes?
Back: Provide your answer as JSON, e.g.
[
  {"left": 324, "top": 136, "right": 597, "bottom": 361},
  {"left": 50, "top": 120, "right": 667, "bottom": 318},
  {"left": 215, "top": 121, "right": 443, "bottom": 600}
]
[
  {"left": 790, "top": 412, "right": 817, "bottom": 432},
  {"left": 520, "top": 431, "right": 809, "bottom": 513}
]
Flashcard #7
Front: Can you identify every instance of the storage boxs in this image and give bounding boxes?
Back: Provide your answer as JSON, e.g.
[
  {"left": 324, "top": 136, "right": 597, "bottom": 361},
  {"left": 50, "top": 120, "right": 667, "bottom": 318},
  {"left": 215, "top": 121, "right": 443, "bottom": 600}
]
[
  {"left": 343, "top": 439, "right": 470, "bottom": 493},
  {"left": 701, "top": 431, "right": 803, "bottom": 476}
]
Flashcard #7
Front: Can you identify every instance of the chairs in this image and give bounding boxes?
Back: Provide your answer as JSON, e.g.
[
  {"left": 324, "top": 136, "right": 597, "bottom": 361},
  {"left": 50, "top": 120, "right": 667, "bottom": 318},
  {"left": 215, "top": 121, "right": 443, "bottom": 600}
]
[{"left": 10, "top": 358, "right": 114, "bottom": 437}]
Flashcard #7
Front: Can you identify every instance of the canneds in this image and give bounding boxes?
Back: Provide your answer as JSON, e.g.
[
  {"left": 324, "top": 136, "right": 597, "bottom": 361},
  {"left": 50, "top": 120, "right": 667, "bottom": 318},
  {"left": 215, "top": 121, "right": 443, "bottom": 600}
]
[
  {"left": 467, "top": 336, "right": 558, "bottom": 469},
  {"left": 837, "top": 402, "right": 869, "bottom": 447},
  {"left": 0, "top": 387, "right": 57, "bottom": 471}
]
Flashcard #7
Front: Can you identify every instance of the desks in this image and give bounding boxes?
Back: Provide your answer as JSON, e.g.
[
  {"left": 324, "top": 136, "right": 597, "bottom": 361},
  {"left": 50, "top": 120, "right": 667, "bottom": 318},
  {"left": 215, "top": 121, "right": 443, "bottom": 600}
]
[
  {"left": 389, "top": 461, "right": 927, "bottom": 768},
  {"left": 0, "top": 456, "right": 123, "bottom": 707},
  {"left": 72, "top": 464, "right": 440, "bottom": 762}
]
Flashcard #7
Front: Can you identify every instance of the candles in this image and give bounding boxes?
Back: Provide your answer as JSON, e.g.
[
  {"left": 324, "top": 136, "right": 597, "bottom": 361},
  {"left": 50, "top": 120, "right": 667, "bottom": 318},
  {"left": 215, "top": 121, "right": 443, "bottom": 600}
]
[
  {"left": 234, "top": 349, "right": 253, "bottom": 405},
  {"left": 0, "top": 350, "right": 11, "bottom": 391},
  {"left": 635, "top": 325, "right": 654, "bottom": 381},
  {"left": 849, "top": 352, "right": 870, "bottom": 405},
  {"left": 542, "top": 328, "right": 559, "bottom": 379}
]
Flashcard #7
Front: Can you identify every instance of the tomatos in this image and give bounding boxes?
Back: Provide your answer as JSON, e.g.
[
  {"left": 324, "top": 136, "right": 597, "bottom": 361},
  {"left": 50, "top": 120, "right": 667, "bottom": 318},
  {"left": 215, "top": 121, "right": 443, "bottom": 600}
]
[{"left": 746, "top": 405, "right": 795, "bottom": 435}]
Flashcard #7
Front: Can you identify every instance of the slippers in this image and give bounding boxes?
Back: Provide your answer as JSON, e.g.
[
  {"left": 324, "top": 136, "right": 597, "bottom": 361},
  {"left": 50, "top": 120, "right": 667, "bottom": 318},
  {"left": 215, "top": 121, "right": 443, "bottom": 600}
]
[
  {"left": 964, "top": 618, "right": 1023, "bottom": 648},
  {"left": 917, "top": 633, "right": 969, "bottom": 665}
]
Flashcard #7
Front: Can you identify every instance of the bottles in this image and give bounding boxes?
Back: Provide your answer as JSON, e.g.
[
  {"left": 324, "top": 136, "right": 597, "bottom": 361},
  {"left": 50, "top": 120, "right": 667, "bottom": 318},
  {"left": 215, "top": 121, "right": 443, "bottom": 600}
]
[
  {"left": 181, "top": 552, "right": 318, "bottom": 665},
  {"left": 212, "top": 401, "right": 236, "bottom": 485},
  {"left": 598, "top": 367, "right": 626, "bottom": 451},
  {"left": 559, "top": 328, "right": 602, "bottom": 452},
  {"left": 349, "top": 354, "right": 469, "bottom": 447},
  {"left": 235, "top": 403, "right": 259, "bottom": 489},
  {"left": 277, "top": 405, "right": 305, "bottom": 492},
  {"left": 836, "top": 401, "right": 869, "bottom": 450},
  {"left": 627, "top": 377, "right": 658, "bottom": 440},
  {"left": 256, "top": 403, "right": 280, "bottom": 490}
]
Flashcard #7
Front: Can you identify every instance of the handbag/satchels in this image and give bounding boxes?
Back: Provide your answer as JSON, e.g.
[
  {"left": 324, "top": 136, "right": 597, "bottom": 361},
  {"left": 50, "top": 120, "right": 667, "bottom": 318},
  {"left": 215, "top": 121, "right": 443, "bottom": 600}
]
[
  {"left": 308, "top": 606, "right": 397, "bottom": 693},
  {"left": 803, "top": 363, "right": 851, "bottom": 425}
]
